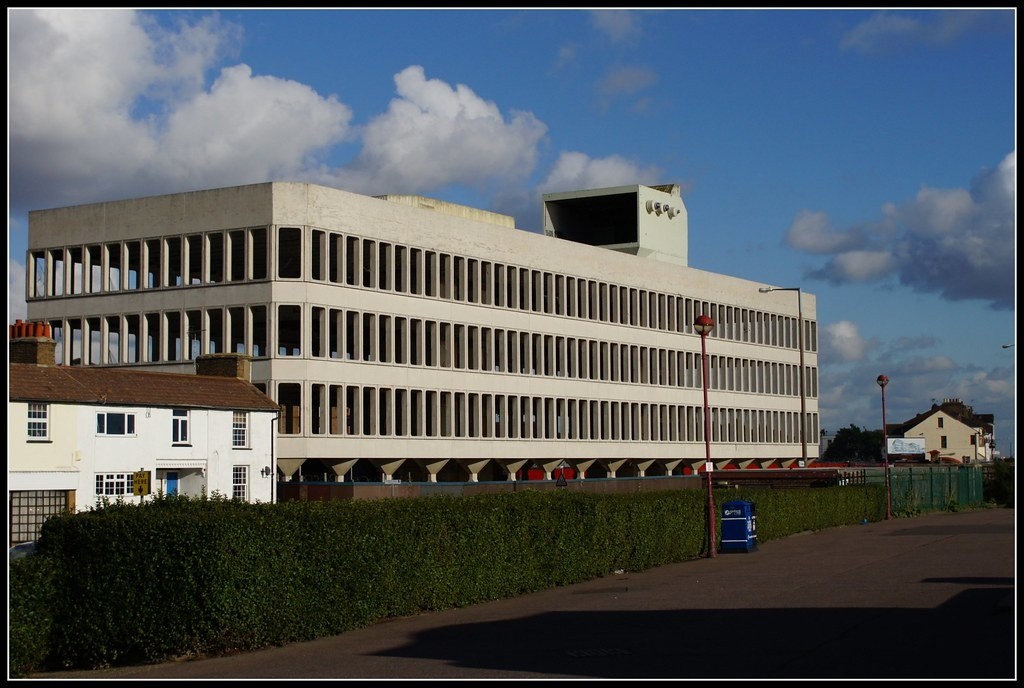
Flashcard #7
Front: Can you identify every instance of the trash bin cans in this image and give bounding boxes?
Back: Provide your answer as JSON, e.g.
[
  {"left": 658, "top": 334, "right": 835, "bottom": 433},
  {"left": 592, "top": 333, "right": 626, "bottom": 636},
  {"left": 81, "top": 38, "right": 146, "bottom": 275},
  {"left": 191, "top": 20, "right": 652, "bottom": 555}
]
[{"left": 720, "top": 500, "right": 757, "bottom": 553}]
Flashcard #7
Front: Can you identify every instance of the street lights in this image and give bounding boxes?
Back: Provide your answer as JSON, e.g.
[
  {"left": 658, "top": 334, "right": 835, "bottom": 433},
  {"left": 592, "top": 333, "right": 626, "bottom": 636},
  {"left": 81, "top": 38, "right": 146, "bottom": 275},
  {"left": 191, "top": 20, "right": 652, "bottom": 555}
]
[
  {"left": 692, "top": 314, "right": 717, "bottom": 559},
  {"left": 876, "top": 375, "right": 893, "bottom": 520},
  {"left": 758, "top": 286, "right": 808, "bottom": 468}
]
